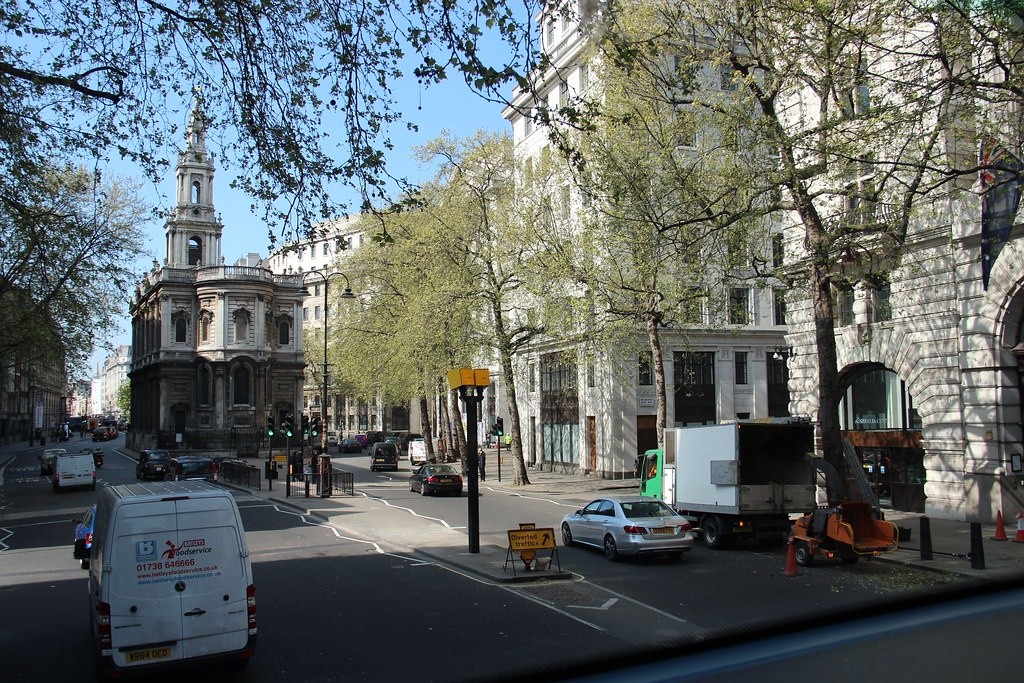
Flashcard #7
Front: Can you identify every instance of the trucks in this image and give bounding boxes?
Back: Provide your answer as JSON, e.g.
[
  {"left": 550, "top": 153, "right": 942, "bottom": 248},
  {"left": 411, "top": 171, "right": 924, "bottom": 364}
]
[{"left": 633, "top": 416, "right": 816, "bottom": 549}]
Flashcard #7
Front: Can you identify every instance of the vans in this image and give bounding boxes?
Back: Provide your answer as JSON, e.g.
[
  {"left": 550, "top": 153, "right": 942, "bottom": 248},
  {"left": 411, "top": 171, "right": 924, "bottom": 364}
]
[
  {"left": 73, "top": 482, "right": 259, "bottom": 683},
  {"left": 48, "top": 453, "right": 96, "bottom": 493}
]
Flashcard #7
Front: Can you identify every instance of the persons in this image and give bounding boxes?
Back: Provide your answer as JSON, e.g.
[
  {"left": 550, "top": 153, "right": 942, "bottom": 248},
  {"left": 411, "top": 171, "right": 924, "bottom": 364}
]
[
  {"left": 504, "top": 433, "right": 511, "bottom": 451},
  {"left": 288, "top": 451, "right": 303, "bottom": 482},
  {"left": 95, "top": 447, "right": 102, "bottom": 454},
  {"left": 88, "top": 425, "right": 94, "bottom": 438},
  {"left": 49, "top": 429, "right": 67, "bottom": 443},
  {"left": 478, "top": 448, "right": 486, "bottom": 481}
]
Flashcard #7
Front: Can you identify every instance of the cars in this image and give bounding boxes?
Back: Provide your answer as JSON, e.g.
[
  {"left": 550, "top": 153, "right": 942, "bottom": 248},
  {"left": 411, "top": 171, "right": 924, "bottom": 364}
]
[
  {"left": 327, "top": 430, "right": 425, "bottom": 460},
  {"left": 136, "top": 450, "right": 171, "bottom": 480},
  {"left": 72, "top": 504, "right": 98, "bottom": 569},
  {"left": 368, "top": 442, "right": 400, "bottom": 472},
  {"left": 38, "top": 449, "right": 67, "bottom": 476},
  {"left": 409, "top": 464, "right": 463, "bottom": 497},
  {"left": 91, "top": 414, "right": 130, "bottom": 443},
  {"left": 162, "top": 456, "right": 218, "bottom": 485},
  {"left": 560, "top": 496, "right": 693, "bottom": 561}
]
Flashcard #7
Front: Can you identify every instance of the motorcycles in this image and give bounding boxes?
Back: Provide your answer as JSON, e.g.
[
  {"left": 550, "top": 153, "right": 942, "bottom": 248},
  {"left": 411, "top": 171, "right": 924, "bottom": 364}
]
[{"left": 93, "top": 452, "right": 104, "bottom": 468}]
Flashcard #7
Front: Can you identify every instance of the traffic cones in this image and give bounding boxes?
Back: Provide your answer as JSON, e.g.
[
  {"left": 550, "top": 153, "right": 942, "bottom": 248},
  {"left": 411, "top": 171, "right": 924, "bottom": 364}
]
[
  {"left": 1011, "top": 512, "right": 1024, "bottom": 543},
  {"left": 990, "top": 509, "right": 1009, "bottom": 541},
  {"left": 781, "top": 536, "right": 803, "bottom": 577}
]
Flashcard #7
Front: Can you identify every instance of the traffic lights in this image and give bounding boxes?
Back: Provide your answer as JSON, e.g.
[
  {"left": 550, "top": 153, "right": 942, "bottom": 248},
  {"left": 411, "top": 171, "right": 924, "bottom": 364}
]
[
  {"left": 267, "top": 413, "right": 318, "bottom": 438},
  {"left": 489, "top": 418, "right": 503, "bottom": 436}
]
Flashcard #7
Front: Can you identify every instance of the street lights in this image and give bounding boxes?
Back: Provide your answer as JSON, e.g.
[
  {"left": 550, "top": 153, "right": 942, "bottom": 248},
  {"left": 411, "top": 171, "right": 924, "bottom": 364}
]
[{"left": 296, "top": 270, "right": 356, "bottom": 499}]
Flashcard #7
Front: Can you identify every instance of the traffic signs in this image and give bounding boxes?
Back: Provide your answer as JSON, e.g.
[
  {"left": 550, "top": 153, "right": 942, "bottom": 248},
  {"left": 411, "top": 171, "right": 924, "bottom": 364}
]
[{"left": 507, "top": 528, "right": 557, "bottom": 551}]
